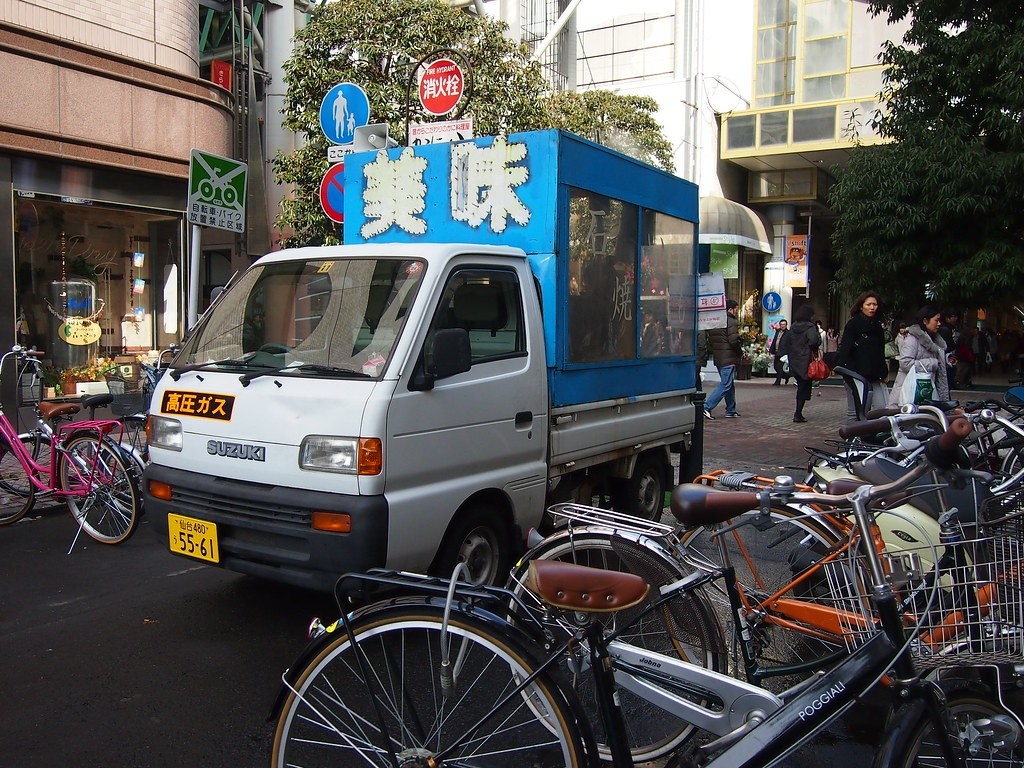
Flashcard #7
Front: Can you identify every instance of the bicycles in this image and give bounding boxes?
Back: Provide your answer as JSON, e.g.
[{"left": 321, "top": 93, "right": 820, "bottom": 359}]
[
  {"left": 261, "top": 361, "right": 1024, "bottom": 767},
  {"left": 0, "top": 343, "right": 181, "bottom": 555}
]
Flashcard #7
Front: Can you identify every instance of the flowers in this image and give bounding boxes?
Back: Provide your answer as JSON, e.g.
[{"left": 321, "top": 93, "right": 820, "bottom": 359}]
[
  {"left": 753, "top": 354, "right": 772, "bottom": 368},
  {"left": 736, "top": 361, "right": 752, "bottom": 380},
  {"left": 738, "top": 321, "right": 768, "bottom": 343},
  {"left": 740, "top": 344, "right": 756, "bottom": 360},
  {"left": 70, "top": 357, "right": 120, "bottom": 380}
]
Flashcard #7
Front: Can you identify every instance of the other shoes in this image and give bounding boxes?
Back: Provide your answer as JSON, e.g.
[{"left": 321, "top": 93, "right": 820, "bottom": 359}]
[
  {"left": 793, "top": 415, "right": 808, "bottom": 423},
  {"left": 725, "top": 409, "right": 742, "bottom": 418},
  {"left": 812, "top": 381, "right": 820, "bottom": 387},
  {"left": 703, "top": 409, "right": 715, "bottom": 420},
  {"left": 772, "top": 382, "right": 780, "bottom": 385},
  {"left": 785, "top": 376, "right": 790, "bottom": 384}
]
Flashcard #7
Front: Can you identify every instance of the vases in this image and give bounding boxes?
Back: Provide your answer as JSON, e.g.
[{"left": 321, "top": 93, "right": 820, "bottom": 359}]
[
  {"left": 75, "top": 381, "right": 110, "bottom": 397},
  {"left": 758, "top": 368, "right": 767, "bottom": 378}
]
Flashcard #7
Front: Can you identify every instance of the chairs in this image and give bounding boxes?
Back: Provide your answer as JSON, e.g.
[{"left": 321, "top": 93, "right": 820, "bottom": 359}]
[
  {"left": 452, "top": 283, "right": 515, "bottom": 357},
  {"left": 351, "top": 284, "right": 400, "bottom": 352}
]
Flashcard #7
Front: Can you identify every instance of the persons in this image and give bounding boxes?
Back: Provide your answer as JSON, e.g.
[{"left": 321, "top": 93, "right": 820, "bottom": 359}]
[
  {"left": 704, "top": 300, "right": 746, "bottom": 419},
  {"left": 640, "top": 311, "right": 671, "bottom": 355},
  {"left": 815, "top": 320, "right": 842, "bottom": 375},
  {"left": 769, "top": 320, "right": 790, "bottom": 385},
  {"left": 881, "top": 321, "right": 907, "bottom": 370},
  {"left": 841, "top": 291, "right": 889, "bottom": 444},
  {"left": 787, "top": 306, "right": 819, "bottom": 421},
  {"left": 679, "top": 320, "right": 708, "bottom": 390},
  {"left": 939, "top": 307, "right": 1024, "bottom": 401},
  {"left": 886, "top": 305, "right": 949, "bottom": 404}
]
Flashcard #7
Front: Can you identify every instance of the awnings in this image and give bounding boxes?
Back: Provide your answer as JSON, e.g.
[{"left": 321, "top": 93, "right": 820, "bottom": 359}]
[{"left": 654, "top": 195, "right": 774, "bottom": 254}]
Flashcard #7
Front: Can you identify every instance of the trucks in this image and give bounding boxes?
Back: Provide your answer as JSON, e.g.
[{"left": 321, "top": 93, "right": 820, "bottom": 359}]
[{"left": 133, "top": 124, "right": 700, "bottom": 610}]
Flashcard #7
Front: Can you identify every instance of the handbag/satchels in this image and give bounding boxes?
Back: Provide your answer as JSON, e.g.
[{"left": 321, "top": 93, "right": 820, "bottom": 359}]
[
  {"left": 985, "top": 351, "right": 992, "bottom": 364},
  {"left": 868, "top": 379, "right": 890, "bottom": 410},
  {"left": 898, "top": 359, "right": 941, "bottom": 407},
  {"left": 808, "top": 347, "right": 830, "bottom": 381},
  {"left": 885, "top": 330, "right": 900, "bottom": 358}
]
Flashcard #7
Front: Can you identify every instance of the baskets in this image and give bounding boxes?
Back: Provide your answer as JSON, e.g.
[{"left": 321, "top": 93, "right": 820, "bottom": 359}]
[
  {"left": 820, "top": 536, "right": 1024, "bottom": 672},
  {"left": 979, "top": 486, "right": 1024, "bottom": 624},
  {"left": 102, "top": 362, "right": 156, "bottom": 416}
]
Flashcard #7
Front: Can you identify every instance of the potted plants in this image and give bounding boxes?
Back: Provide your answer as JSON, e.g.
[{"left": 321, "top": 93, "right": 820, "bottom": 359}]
[
  {"left": 59, "top": 368, "right": 76, "bottom": 395},
  {"left": 42, "top": 367, "right": 62, "bottom": 398}
]
